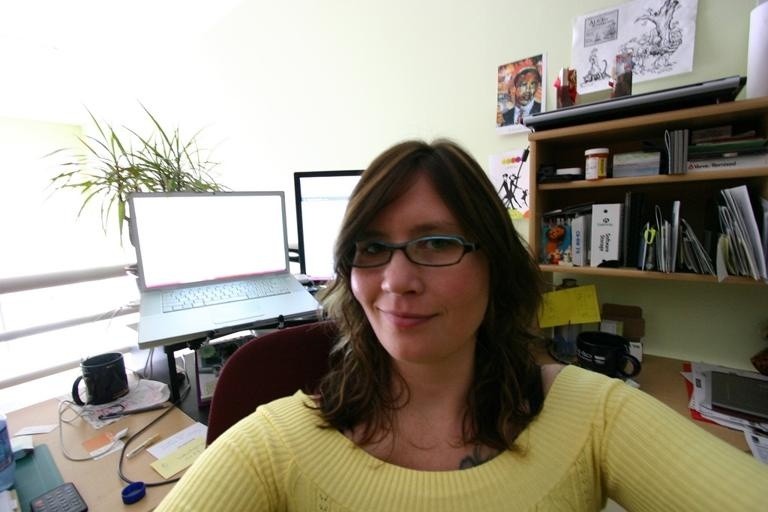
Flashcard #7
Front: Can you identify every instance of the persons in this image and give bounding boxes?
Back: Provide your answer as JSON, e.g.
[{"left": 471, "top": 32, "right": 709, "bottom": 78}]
[
  {"left": 148, "top": 133, "right": 767, "bottom": 511},
  {"left": 495, "top": 66, "right": 542, "bottom": 125}
]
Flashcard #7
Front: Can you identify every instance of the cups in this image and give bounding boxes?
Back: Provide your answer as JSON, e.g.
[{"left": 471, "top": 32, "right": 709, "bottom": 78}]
[
  {"left": 72, "top": 354, "right": 129, "bottom": 406},
  {"left": 576, "top": 329, "right": 640, "bottom": 382}
]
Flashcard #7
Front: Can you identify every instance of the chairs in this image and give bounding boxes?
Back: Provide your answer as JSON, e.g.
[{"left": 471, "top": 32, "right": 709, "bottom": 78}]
[{"left": 206, "top": 320, "right": 347, "bottom": 454}]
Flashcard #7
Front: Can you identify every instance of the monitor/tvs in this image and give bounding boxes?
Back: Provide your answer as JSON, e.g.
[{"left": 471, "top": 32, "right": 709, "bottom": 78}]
[{"left": 294, "top": 170, "right": 364, "bottom": 285}]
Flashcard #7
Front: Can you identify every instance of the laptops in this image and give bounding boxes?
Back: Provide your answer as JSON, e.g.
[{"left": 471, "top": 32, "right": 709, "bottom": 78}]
[{"left": 127, "top": 190, "right": 325, "bottom": 350}]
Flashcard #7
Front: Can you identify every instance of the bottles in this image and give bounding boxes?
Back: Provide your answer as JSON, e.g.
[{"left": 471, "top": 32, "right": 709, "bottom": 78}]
[
  {"left": 585, "top": 148, "right": 609, "bottom": 180},
  {"left": 0, "top": 414, "right": 17, "bottom": 492},
  {"left": 556, "top": 278, "right": 585, "bottom": 359}
]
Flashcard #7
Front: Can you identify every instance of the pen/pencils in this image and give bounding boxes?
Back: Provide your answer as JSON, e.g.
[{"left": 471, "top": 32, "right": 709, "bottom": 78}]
[
  {"left": 126, "top": 433, "right": 161, "bottom": 457},
  {"left": 98, "top": 404, "right": 169, "bottom": 420}
]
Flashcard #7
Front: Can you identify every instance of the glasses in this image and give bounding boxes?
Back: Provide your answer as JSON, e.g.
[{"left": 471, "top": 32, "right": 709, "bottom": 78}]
[{"left": 340, "top": 234, "right": 496, "bottom": 267}]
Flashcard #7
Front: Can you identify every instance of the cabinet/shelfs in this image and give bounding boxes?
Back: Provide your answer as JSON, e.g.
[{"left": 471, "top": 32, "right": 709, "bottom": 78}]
[
  {"left": 4, "top": 372, "right": 209, "bottom": 512},
  {"left": 528, "top": 98, "right": 768, "bottom": 462}
]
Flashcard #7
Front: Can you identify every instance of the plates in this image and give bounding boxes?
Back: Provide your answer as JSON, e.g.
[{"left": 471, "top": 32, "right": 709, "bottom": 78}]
[{"left": 80, "top": 378, "right": 172, "bottom": 418}]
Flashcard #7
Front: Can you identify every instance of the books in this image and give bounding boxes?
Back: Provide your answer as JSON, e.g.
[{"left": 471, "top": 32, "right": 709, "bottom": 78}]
[
  {"left": 534, "top": 116, "right": 768, "bottom": 279},
  {"left": 704, "top": 368, "right": 767, "bottom": 422}
]
